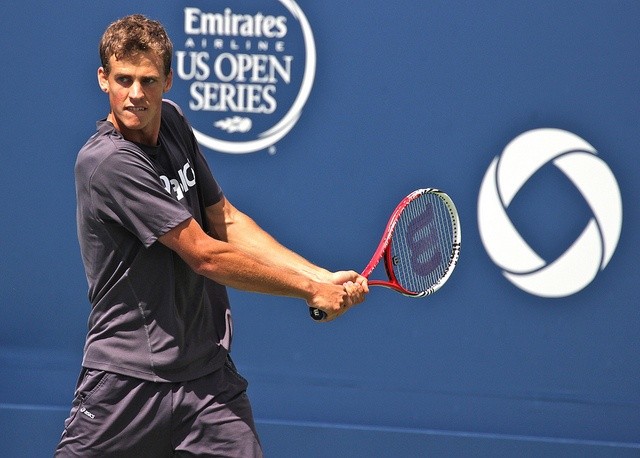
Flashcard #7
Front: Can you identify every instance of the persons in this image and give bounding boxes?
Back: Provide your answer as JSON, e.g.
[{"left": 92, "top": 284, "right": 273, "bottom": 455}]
[{"left": 58, "top": 14, "right": 370, "bottom": 458}]
